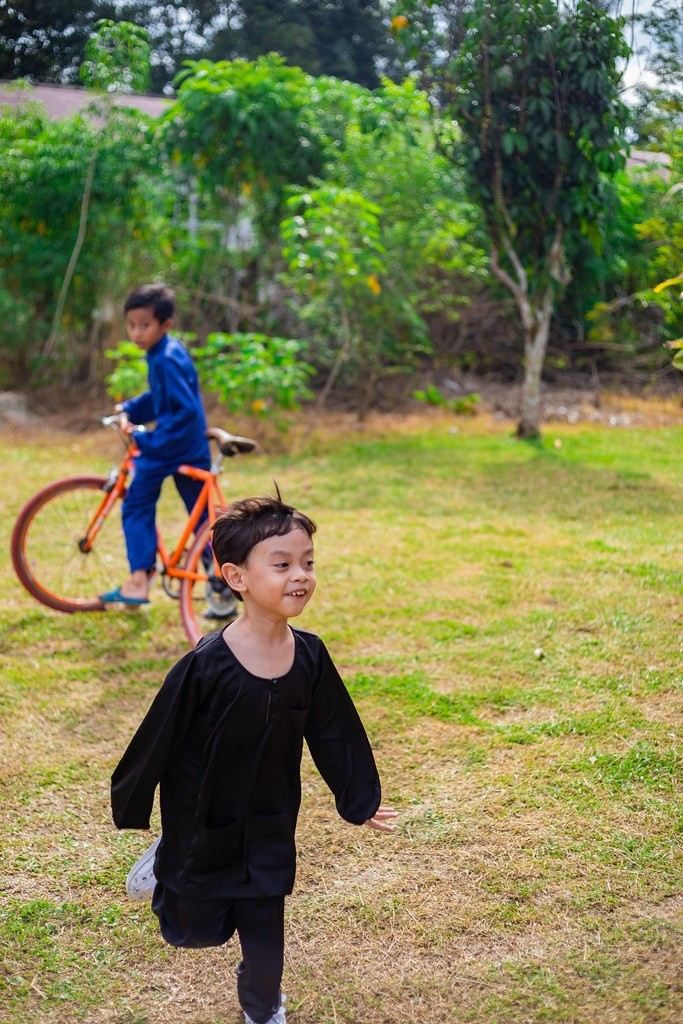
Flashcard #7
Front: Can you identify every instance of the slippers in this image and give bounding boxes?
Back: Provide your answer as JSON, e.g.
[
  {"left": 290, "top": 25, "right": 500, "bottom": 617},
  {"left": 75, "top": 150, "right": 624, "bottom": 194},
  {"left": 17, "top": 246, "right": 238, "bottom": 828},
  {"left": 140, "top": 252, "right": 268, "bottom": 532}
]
[
  {"left": 99, "top": 585, "right": 148, "bottom": 604},
  {"left": 204, "top": 610, "right": 238, "bottom": 619}
]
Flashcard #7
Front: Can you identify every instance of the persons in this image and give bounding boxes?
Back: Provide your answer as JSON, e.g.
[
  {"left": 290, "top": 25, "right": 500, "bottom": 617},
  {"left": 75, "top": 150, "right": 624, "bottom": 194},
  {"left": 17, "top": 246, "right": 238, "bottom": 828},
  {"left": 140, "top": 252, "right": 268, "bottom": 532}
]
[
  {"left": 98, "top": 284, "right": 241, "bottom": 620},
  {"left": 110, "top": 479, "right": 400, "bottom": 1024}
]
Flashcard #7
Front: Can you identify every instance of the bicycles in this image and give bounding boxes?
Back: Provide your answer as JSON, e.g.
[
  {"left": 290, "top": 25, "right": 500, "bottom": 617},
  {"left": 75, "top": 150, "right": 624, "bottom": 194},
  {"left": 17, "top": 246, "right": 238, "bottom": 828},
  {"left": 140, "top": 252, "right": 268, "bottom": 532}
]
[{"left": 10, "top": 412, "right": 270, "bottom": 651}]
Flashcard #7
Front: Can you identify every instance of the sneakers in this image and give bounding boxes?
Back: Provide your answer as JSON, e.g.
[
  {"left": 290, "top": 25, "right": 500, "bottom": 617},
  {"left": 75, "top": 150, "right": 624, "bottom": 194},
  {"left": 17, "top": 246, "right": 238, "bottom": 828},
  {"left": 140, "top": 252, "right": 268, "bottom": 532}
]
[
  {"left": 244, "top": 995, "right": 287, "bottom": 1024},
  {"left": 126, "top": 835, "right": 162, "bottom": 902}
]
